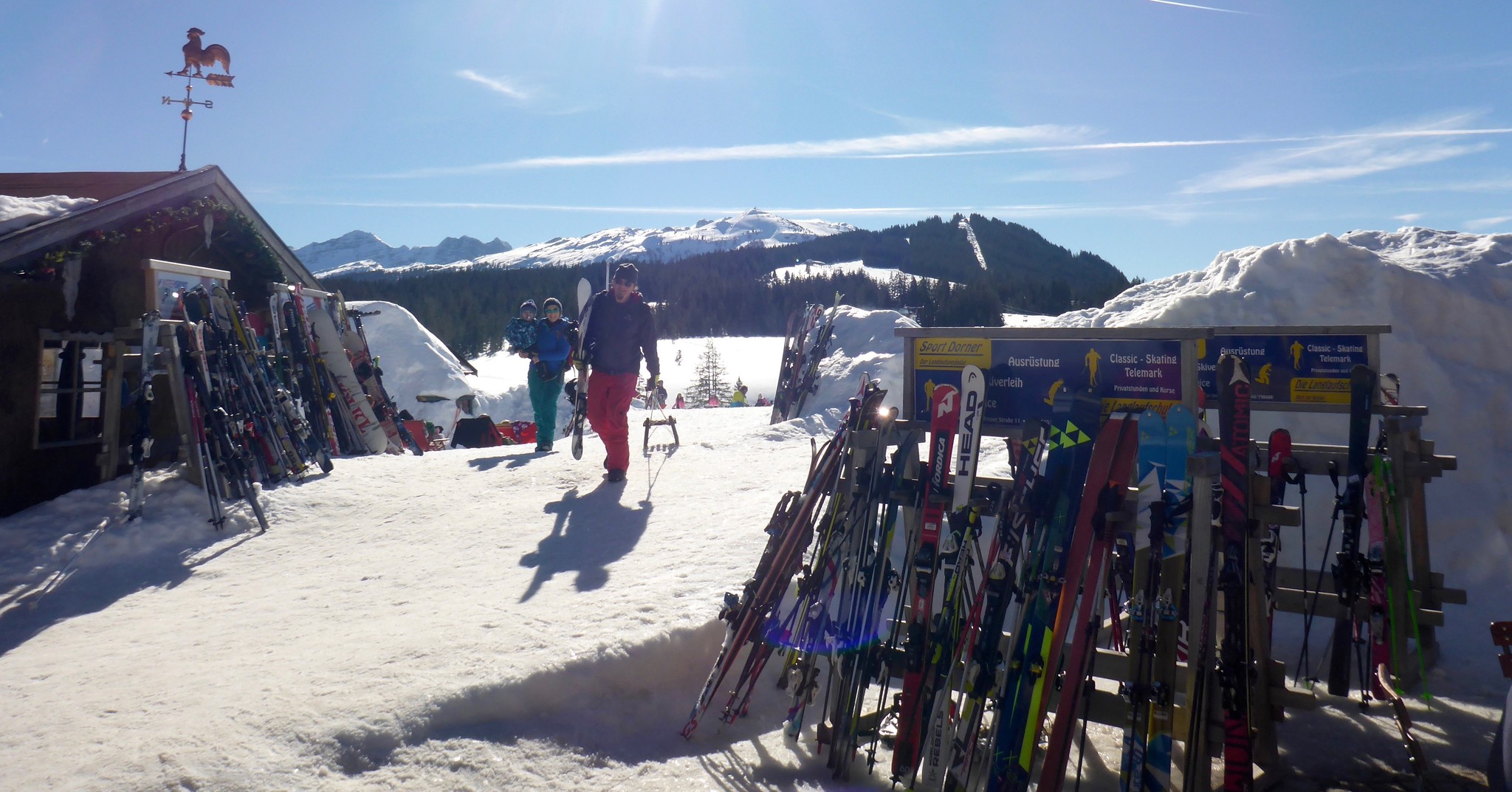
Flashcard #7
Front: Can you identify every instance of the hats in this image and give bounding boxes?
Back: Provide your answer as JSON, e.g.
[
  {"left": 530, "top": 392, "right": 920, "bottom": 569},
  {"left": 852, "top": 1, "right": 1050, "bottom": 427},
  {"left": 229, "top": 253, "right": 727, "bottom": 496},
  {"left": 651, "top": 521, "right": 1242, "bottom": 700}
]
[
  {"left": 758, "top": 393, "right": 763, "bottom": 399},
  {"left": 613, "top": 263, "right": 638, "bottom": 284},
  {"left": 711, "top": 392, "right": 716, "bottom": 396},
  {"left": 543, "top": 298, "right": 562, "bottom": 316},
  {"left": 520, "top": 300, "right": 537, "bottom": 317}
]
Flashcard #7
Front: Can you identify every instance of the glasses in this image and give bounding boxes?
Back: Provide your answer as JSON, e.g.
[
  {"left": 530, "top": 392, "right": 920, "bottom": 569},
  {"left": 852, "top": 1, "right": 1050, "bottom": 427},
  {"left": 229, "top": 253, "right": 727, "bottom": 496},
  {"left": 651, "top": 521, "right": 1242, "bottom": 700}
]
[
  {"left": 545, "top": 308, "right": 560, "bottom": 313},
  {"left": 615, "top": 278, "right": 632, "bottom": 287}
]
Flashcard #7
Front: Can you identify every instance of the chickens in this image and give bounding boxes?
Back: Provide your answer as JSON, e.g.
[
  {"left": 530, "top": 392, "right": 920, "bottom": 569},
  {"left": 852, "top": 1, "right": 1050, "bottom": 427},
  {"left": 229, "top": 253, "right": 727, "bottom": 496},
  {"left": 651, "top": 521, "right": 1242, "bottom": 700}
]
[{"left": 177, "top": 28, "right": 230, "bottom": 77}]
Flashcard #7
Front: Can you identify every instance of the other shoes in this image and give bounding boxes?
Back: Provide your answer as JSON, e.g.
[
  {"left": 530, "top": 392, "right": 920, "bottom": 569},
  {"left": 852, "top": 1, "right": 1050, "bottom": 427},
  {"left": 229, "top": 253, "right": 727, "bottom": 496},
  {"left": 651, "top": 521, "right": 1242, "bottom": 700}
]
[
  {"left": 608, "top": 469, "right": 625, "bottom": 482},
  {"left": 604, "top": 455, "right": 608, "bottom": 467},
  {"left": 543, "top": 441, "right": 552, "bottom": 450},
  {"left": 542, "top": 371, "right": 557, "bottom": 382}
]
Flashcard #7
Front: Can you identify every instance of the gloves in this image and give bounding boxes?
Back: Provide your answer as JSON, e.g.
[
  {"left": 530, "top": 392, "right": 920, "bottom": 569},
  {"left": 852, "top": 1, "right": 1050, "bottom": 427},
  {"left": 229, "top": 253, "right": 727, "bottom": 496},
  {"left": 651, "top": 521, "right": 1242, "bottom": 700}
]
[
  {"left": 645, "top": 373, "right": 660, "bottom": 392},
  {"left": 572, "top": 346, "right": 591, "bottom": 370}
]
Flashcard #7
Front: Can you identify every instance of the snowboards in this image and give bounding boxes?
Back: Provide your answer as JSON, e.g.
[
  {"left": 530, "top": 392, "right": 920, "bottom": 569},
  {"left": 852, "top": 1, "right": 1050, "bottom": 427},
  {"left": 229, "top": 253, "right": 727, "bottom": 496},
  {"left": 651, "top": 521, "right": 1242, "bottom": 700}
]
[
  {"left": 267, "top": 281, "right": 425, "bottom": 456},
  {"left": 571, "top": 276, "right": 593, "bottom": 460}
]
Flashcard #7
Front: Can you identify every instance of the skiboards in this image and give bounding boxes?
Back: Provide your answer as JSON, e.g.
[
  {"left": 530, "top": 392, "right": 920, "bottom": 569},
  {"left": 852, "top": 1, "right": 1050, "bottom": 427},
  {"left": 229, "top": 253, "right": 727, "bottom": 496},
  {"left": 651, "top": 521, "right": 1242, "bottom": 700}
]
[
  {"left": 126, "top": 282, "right": 333, "bottom": 531},
  {"left": 672, "top": 353, "right": 1433, "bottom": 792}
]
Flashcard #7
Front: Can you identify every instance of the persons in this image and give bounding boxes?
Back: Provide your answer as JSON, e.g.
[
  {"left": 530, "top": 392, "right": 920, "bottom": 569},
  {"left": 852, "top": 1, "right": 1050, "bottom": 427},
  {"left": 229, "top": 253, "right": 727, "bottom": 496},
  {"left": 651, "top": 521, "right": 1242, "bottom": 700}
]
[
  {"left": 572, "top": 263, "right": 660, "bottom": 481},
  {"left": 653, "top": 381, "right": 771, "bottom": 409},
  {"left": 505, "top": 300, "right": 558, "bottom": 383},
  {"left": 518, "top": 297, "right": 574, "bottom": 452}
]
